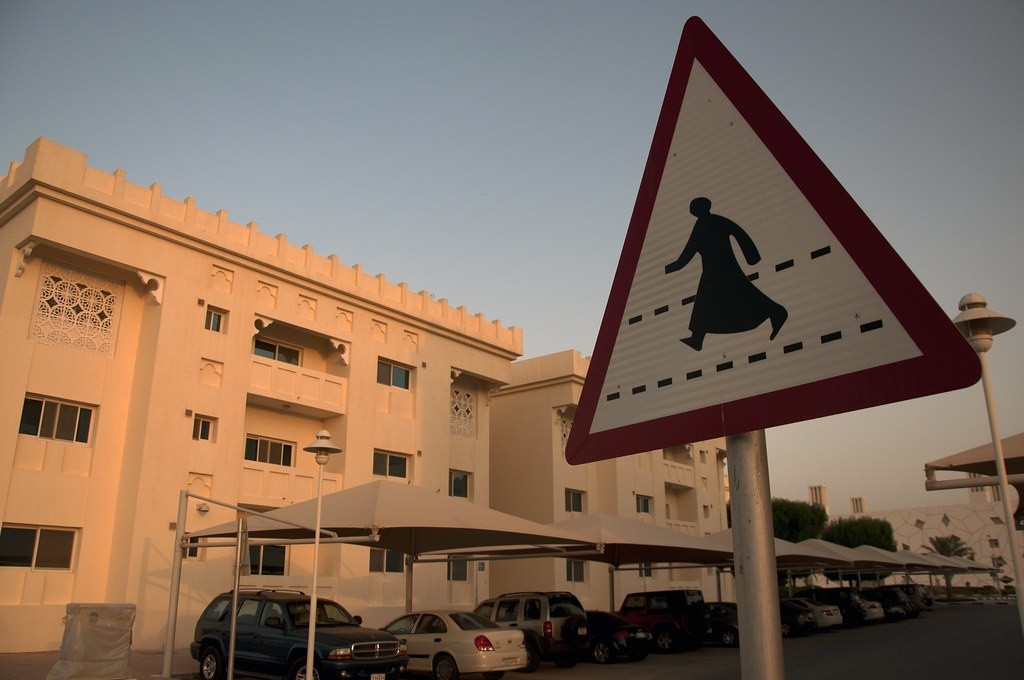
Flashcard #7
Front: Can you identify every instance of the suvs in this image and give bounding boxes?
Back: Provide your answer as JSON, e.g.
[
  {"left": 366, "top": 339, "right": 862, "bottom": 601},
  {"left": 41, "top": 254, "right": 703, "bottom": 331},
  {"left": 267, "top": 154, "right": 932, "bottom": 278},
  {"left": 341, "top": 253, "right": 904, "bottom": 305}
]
[
  {"left": 616, "top": 589, "right": 704, "bottom": 655},
  {"left": 191, "top": 588, "right": 409, "bottom": 680},
  {"left": 473, "top": 591, "right": 590, "bottom": 673}
]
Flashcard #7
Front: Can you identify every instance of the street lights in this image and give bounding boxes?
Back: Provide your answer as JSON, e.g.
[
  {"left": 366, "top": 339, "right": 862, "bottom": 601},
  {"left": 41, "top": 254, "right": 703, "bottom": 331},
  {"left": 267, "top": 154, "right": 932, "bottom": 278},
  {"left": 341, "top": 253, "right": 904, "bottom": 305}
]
[
  {"left": 950, "top": 294, "right": 1024, "bottom": 630},
  {"left": 303, "top": 430, "right": 343, "bottom": 680}
]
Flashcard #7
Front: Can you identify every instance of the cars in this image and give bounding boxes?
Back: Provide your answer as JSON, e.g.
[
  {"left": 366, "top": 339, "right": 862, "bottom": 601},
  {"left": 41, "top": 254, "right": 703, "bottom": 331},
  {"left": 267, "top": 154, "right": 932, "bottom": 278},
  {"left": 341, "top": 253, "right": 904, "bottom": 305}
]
[
  {"left": 586, "top": 610, "right": 653, "bottom": 665},
  {"left": 793, "top": 584, "right": 934, "bottom": 628},
  {"left": 705, "top": 602, "right": 814, "bottom": 648},
  {"left": 383, "top": 608, "right": 529, "bottom": 680},
  {"left": 784, "top": 598, "right": 843, "bottom": 633}
]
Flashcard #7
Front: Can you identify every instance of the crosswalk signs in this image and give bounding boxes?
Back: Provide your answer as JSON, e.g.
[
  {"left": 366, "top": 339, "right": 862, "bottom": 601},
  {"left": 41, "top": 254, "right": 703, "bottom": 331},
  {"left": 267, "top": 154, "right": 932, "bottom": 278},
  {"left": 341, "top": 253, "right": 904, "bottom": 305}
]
[{"left": 565, "top": 14, "right": 983, "bottom": 470}]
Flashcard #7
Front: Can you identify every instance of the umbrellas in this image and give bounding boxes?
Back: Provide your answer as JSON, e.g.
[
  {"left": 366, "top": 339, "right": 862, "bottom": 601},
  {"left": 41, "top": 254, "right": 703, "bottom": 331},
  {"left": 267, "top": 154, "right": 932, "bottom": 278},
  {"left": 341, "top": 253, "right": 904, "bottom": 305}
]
[{"left": 162, "top": 477, "right": 1006, "bottom": 680}]
